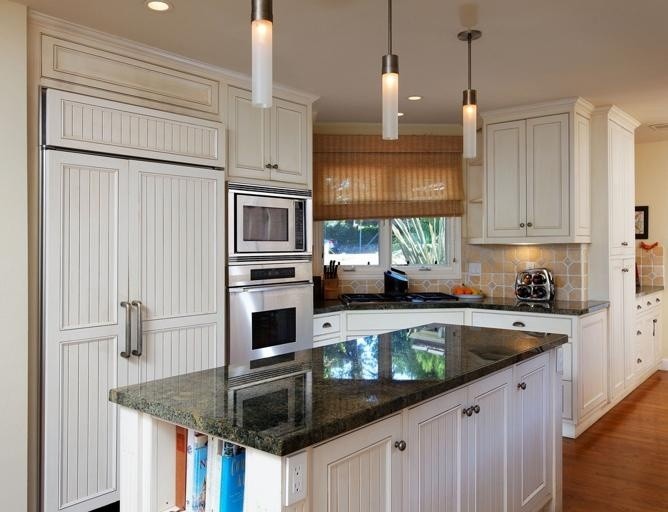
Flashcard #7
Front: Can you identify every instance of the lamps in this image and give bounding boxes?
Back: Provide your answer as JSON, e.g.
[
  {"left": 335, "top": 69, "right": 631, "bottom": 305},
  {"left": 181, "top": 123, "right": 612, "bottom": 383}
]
[
  {"left": 382, "top": 0, "right": 399, "bottom": 141},
  {"left": 251, "top": 1, "right": 272, "bottom": 109},
  {"left": 457, "top": 29, "right": 482, "bottom": 159}
]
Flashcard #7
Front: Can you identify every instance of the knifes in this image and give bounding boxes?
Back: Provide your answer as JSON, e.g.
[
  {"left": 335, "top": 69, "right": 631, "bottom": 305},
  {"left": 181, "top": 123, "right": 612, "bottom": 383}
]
[{"left": 323, "top": 260, "right": 341, "bottom": 279}]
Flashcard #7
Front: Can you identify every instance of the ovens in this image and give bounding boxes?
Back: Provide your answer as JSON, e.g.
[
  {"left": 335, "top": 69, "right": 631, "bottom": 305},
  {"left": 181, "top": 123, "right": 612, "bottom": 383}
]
[
  {"left": 226, "top": 349, "right": 314, "bottom": 437},
  {"left": 226, "top": 181, "right": 315, "bottom": 363}
]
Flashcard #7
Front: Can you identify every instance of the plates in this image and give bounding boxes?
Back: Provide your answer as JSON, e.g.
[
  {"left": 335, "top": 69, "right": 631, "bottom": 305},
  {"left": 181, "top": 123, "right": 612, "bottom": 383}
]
[{"left": 452, "top": 294, "right": 485, "bottom": 299}]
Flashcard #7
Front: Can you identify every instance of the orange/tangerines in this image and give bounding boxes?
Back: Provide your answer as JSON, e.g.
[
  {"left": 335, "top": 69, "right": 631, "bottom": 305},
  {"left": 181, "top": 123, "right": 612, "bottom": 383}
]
[
  {"left": 462, "top": 287, "right": 472, "bottom": 295},
  {"left": 452, "top": 288, "right": 464, "bottom": 295}
]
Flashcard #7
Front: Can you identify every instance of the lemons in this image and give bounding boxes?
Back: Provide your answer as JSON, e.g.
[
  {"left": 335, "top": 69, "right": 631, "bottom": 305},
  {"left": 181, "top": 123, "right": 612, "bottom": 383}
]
[{"left": 470, "top": 286, "right": 479, "bottom": 295}]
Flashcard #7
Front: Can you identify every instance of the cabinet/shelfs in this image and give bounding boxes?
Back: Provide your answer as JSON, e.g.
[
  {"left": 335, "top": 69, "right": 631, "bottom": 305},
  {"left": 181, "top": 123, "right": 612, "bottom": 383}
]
[
  {"left": 311, "top": 413, "right": 407, "bottom": 512},
  {"left": 226, "top": 83, "right": 309, "bottom": 184},
  {"left": 471, "top": 309, "right": 574, "bottom": 438},
  {"left": 586, "top": 103, "right": 636, "bottom": 404},
  {"left": 466, "top": 96, "right": 593, "bottom": 245},
  {"left": 636, "top": 291, "right": 663, "bottom": 380},
  {"left": 512, "top": 350, "right": 553, "bottom": 511},
  {"left": 42, "top": 149, "right": 225, "bottom": 511},
  {"left": 403, "top": 366, "right": 514, "bottom": 512}
]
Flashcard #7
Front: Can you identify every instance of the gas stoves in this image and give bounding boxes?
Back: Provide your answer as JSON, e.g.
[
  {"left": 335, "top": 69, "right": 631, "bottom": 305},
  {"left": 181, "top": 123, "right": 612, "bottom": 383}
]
[{"left": 340, "top": 292, "right": 459, "bottom": 308}]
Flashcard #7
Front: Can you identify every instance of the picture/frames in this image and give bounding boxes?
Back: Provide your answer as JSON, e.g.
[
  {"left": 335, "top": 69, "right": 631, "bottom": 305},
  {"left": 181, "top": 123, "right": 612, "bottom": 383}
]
[{"left": 635, "top": 205, "right": 649, "bottom": 240}]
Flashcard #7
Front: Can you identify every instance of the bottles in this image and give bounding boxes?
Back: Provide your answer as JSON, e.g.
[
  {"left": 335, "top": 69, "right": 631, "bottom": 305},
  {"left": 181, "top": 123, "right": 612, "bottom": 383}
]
[{"left": 635, "top": 262, "right": 640, "bottom": 293}]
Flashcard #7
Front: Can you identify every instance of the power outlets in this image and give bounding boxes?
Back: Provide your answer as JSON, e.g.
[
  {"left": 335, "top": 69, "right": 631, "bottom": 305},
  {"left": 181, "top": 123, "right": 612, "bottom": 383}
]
[{"left": 285, "top": 451, "right": 308, "bottom": 507}]
